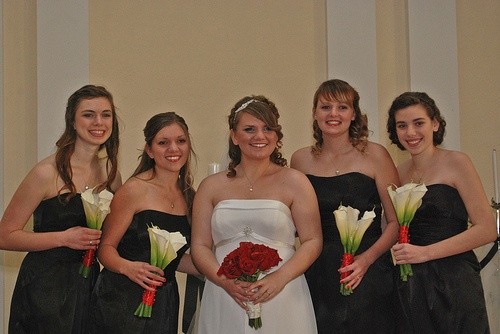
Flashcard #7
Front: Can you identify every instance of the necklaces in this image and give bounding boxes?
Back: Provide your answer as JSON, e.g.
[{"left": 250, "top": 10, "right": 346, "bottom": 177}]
[
  {"left": 318, "top": 149, "right": 357, "bottom": 175},
  {"left": 411, "top": 159, "right": 426, "bottom": 185},
  {"left": 157, "top": 181, "right": 183, "bottom": 209},
  {"left": 240, "top": 164, "right": 271, "bottom": 192},
  {"left": 74, "top": 160, "right": 99, "bottom": 189}
]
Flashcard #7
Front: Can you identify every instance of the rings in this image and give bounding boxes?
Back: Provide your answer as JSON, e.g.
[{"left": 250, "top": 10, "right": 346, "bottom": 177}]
[
  {"left": 265, "top": 291, "right": 270, "bottom": 296},
  {"left": 89, "top": 240, "right": 92, "bottom": 245}
]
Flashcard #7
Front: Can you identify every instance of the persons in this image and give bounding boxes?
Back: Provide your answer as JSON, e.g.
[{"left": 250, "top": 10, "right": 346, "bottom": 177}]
[
  {"left": 290, "top": 80, "right": 404, "bottom": 334},
  {"left": 382, "top": 90, "right": 498, "bottom": 334},
  {"left": 0, "top": 84, "right": 123, "bottom": 334},
  {"left": 89, "top": 112, "right": 206, "bottom": 334},
  {"left": 188, "top": 94, "right": 323, "bottom": 334}
]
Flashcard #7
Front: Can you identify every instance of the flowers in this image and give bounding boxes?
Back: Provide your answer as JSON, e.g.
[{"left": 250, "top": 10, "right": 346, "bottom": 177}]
[
  {"left": 333, "top": 201, "right": 377, "bottom": 296},
  {"left": 384, "top": 175, "right": 428, "bottom": 282},
  {"left": 78, "top": 188, "right": 112, "bottom": 279},
  {"left": 133, "top": 222, "right": 187, "bottom": 318},
  {"left": 216, "top": 241, "right": 282, "bottom": 329}
]
